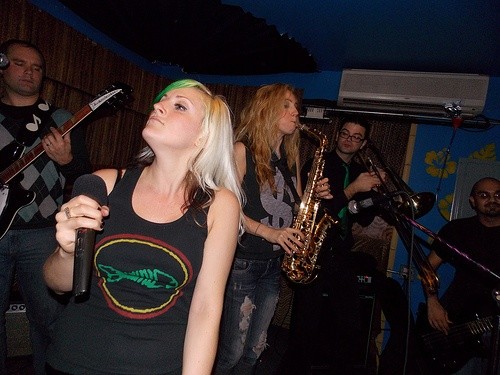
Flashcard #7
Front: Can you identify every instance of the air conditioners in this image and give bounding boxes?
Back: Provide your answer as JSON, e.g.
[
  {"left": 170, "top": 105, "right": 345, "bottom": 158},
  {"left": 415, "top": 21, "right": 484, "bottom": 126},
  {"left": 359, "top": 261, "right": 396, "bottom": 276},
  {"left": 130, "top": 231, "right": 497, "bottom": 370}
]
[{"left": 337, "top": 68, "right": 489, "bottom": 118}]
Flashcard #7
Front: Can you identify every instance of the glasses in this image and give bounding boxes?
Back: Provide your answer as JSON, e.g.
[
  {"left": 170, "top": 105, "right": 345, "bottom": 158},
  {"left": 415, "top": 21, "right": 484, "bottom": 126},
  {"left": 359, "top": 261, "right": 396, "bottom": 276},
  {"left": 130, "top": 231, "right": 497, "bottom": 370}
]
[
  {"left": 472, "top": 192, "right": 500, "bottom": 199},
  {"left": 339, "top": 131, "right": 364, "bottom": 143}
]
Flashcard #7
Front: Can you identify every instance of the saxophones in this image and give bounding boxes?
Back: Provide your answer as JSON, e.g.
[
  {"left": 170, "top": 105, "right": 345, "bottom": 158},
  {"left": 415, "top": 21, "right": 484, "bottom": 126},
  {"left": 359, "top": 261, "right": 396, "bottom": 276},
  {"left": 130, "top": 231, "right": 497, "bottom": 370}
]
[{"left": 278, "top": 120, "right": 339, "bottom": 286}]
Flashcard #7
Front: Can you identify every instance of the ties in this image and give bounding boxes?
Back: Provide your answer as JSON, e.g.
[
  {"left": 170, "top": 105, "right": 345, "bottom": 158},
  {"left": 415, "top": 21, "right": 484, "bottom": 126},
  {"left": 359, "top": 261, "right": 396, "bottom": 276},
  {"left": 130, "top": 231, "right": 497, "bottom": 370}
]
[{"left": 335, "top": 162, "right": 352, "bottom": 224}]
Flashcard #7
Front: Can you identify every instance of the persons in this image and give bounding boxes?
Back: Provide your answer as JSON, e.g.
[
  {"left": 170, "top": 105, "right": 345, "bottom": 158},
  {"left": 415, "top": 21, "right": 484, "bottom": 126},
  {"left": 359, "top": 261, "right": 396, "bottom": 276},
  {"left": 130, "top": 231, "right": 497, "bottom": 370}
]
[
  {"left": 42, "top": 80, "right": 247, "bottom": 375},
  {"left": 0, "top": 39, "right": 90, "bottom": 375},
  {"left": 417, "top": 177, "right": 500, "bottom": 375},
  {"left": 209, "top": 84, "right": 333, "bottom": 375},
  {"left": 279, "top": 113, "right": 386, "bottom": 375}
]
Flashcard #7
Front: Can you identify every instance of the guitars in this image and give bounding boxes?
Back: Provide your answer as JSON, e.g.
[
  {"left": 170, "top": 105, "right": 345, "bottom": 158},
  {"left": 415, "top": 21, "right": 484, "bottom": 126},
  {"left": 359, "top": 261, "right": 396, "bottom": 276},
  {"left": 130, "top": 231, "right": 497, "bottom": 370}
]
[
  {"left": 0, "top": 81, "right": 134, "bottom": 240},
  {"left": 413, "top": 301, "right": 500, "bottom": 375}
]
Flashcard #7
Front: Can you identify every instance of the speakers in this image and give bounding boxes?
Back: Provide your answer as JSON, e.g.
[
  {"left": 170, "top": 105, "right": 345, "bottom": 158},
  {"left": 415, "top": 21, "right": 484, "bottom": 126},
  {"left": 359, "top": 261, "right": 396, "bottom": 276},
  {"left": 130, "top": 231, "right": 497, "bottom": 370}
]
[{"left": 309, "top": 294, "right": 375, "bottom": 369}]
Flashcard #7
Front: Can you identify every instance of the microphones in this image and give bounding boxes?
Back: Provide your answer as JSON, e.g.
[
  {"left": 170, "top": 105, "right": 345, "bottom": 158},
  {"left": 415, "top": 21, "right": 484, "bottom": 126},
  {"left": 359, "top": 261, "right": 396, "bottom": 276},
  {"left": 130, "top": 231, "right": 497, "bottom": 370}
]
[
  {"left": 0, "top": 53, "right": 8, "bottom": 67},
  {"left": 348, "top": 189, "right": 404, "bottom": 215},
  {"left": 71, "top": 174, "right": 108, "bottom": 300}
]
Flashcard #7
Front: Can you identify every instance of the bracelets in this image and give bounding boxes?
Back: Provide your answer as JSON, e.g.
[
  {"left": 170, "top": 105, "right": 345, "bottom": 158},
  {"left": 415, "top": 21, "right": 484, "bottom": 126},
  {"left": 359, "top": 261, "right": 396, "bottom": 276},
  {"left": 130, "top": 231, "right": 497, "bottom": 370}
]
[{"left": 254, "top": 222, "right": 263, "bottom": 237}]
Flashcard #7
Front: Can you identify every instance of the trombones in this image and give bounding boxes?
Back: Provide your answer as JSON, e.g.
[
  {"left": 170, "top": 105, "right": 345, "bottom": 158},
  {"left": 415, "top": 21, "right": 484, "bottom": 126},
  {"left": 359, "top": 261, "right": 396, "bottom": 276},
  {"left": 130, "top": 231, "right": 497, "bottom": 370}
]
[{"left": 356, "top": 137, "right": 442, "bottom": 297}]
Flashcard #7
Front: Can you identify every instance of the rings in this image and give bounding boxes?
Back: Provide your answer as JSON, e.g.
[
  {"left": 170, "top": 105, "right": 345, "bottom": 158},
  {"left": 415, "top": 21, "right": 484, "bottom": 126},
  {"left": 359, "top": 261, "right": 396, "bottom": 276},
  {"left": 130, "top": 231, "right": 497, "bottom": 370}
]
[
  {"left": 47, "top": 142, "right": 52, "bottom": 147},
  {"left": 64, "top": 206, "right": 70, "bottom": 219}
]
